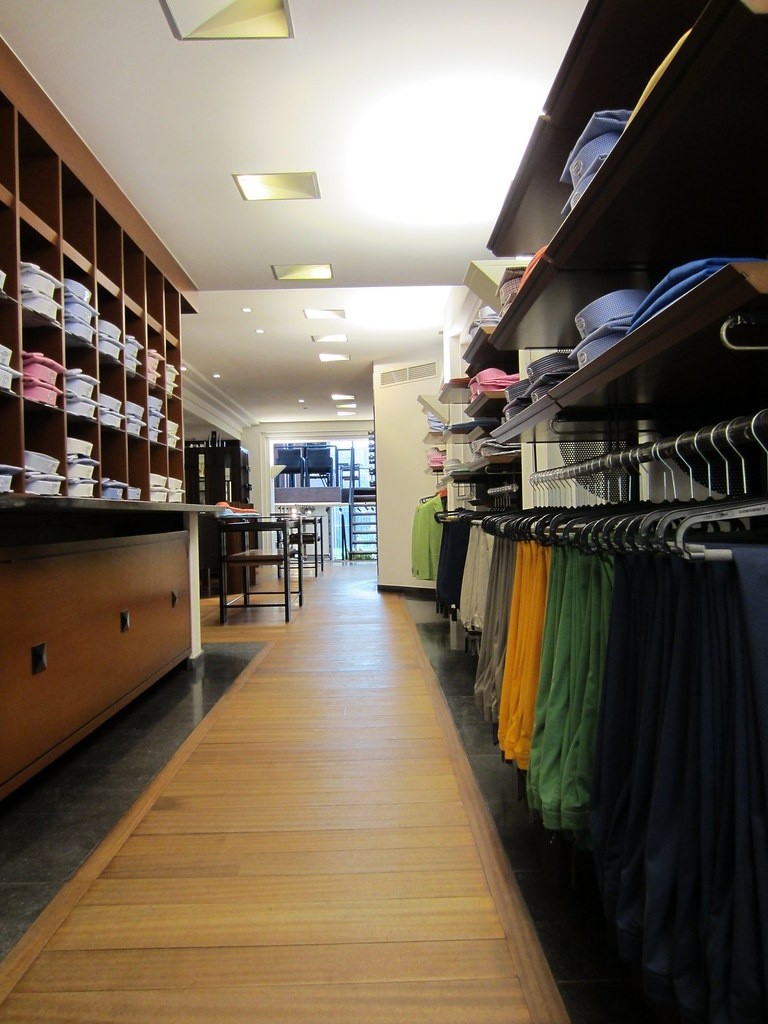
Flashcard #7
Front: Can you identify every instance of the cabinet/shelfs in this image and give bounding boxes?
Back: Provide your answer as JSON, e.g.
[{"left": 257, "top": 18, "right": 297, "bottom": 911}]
[
  {"left": 0, "top": 38, "right": 228, "bottom": 801},
  {"left": 423, "top": 1, "right": 767, "bottom": 483},
  {"left": 276, "top": 516, "right": 323, "bottom": 578},
  {"left": 219, "top": 520, "right": 304, "bottom": 623}
]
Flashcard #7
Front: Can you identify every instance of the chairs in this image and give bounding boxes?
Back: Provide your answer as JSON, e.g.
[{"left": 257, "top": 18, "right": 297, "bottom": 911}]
[
  {"left": 304, "top": 447, "right": 333, "bottom": 488},
  {"left": 276, "top": 448, "right": 305, "bottom": 488}
]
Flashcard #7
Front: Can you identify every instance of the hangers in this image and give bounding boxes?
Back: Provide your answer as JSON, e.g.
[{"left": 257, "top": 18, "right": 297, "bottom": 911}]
[{"left": 420, "top": 409, "right": 768, "bottom": 562}]
